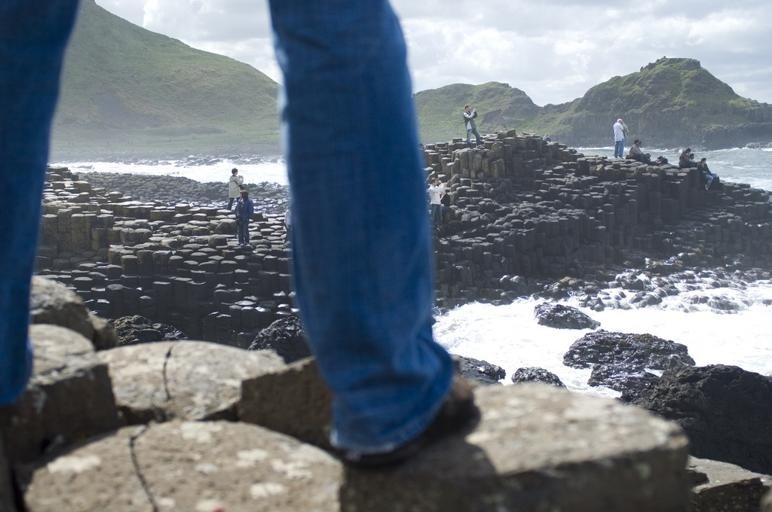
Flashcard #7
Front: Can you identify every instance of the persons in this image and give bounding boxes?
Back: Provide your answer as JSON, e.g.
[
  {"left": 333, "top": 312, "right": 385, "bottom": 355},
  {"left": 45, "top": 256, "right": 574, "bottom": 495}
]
[
  {"left": 426, "top": 178, "right": 445, "bottom": 224},
  {"left": 679, "top": 148, "right": 717, "bottom": 191},
  {"left": 463, "top": 105, "right": 481, "bottom": 147},
  {"left": 284, "top": 208, "right": 292, "bottom": 242},
  {"left": 227, "top": 168, "right": 243, "bottom": 211},
  {"left": 0, "top": 0, "right": 475, "bottom": 471},
  {"left": 613, "top": 118, "right": 651, "bottom": 162},
  {"left": 234, "top": 189, "right": 254, "bottom": 245}
]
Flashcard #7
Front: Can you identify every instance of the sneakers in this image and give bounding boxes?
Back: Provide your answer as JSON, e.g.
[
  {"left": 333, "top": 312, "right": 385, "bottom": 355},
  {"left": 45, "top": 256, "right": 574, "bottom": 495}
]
[{"left": 361, "top": 375, "right": 473, "bottom": 470}]
